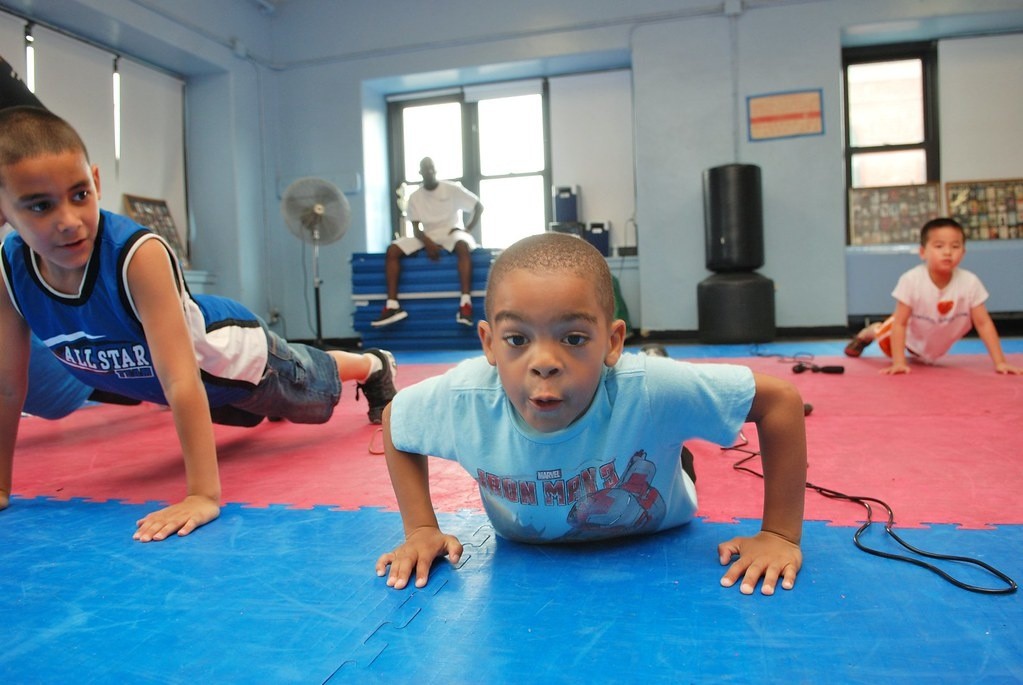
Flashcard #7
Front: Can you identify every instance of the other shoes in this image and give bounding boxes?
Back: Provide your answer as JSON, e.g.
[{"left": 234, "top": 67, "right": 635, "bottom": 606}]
[{"left": 641, "top": 344, "right": 667, "bottom": 358}]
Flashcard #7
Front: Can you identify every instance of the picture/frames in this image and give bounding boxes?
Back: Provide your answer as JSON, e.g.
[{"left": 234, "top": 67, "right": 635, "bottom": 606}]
[{"left": 124, "top": 192, "right": 191, "bottom": 270}]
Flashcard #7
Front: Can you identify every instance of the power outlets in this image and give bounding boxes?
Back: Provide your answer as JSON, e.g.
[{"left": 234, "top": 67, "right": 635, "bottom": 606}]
[{"left": 272, "top": 308, "right": 279, "bottom": 322}]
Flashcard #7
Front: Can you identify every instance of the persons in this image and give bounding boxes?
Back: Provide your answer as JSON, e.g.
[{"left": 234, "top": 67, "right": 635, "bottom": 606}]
[
  {"left": 375, "top": 234, "right": 807, "bottom": 595},
  {"left": 0, "top": 57, "right": 398, "bottom": 542},
  {"left": 844, "top": 218, "right": 1023, "bottom": 375},
  {"left": 371, "top": 157, "right": 484, "bottom": 326}
]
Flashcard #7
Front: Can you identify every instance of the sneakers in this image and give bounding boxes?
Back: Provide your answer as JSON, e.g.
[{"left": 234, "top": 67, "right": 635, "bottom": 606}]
[
  {"left": 844, "top": 334, "right": 874, "bottom": 356},
  {"left": 456, "top": 303, "right": 474, "bottom": 326},
  {"left": 371, "top": 305, "right": 408, "bottom": 327},
  {"left": 356, "top": 346, "right": 398, "bottom": 424},
  {"left": 269, "top": 413, "right": 284, "bottom": 423}
]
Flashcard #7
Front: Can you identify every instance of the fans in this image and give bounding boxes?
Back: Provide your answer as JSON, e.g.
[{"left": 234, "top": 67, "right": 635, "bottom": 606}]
[{"left": 281, "top": 176, "right": 353, "bottom": 354}]
